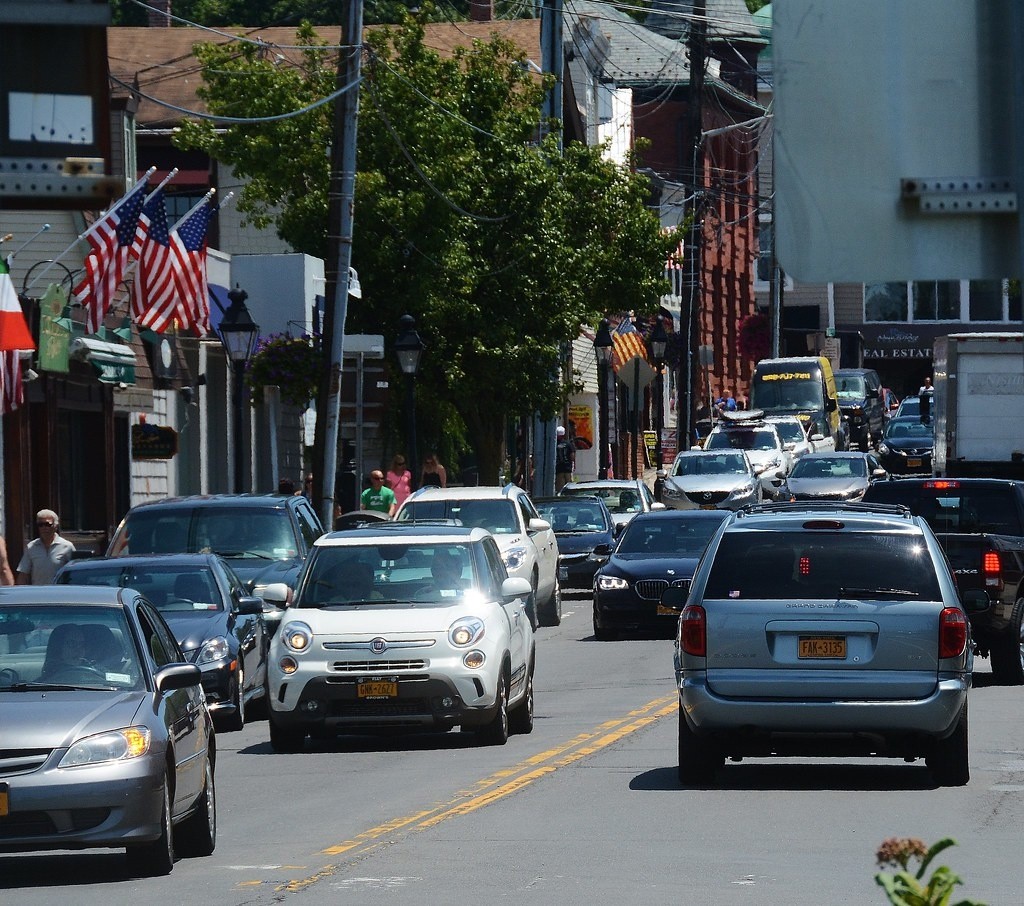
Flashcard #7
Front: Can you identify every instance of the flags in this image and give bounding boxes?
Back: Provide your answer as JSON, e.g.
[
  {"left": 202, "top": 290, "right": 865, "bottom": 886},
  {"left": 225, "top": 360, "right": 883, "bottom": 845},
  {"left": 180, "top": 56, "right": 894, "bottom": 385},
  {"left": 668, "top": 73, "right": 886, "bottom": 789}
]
[
  {"left": 74, "top": 181, "right": 149, "bottom": 336},
  {"left": 130, "top": 189, "right": 175, "bottom": 334},
  {"left": 168, "top": 200, "right": 214, "bottom": 338},
  {"left": 0, "top": 256, "right": 37, "bottom": 415},
  {"left": 611, "top": 318, "right": 648, "bottom": 373}
]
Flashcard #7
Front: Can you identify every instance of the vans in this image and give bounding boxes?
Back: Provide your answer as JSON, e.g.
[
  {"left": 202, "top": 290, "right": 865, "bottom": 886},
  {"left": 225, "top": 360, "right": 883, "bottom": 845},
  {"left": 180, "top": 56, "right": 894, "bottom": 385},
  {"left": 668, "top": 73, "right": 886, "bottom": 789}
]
[{"left": 831, "top": 369, "right": 889, "bottom": 451}]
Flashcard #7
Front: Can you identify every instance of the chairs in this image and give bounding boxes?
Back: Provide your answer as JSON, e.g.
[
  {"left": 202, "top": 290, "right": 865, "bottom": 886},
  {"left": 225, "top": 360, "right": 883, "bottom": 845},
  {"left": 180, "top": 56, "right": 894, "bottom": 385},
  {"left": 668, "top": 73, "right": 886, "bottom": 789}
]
[
  {"left": 175, "top": 574, "right": 203, "bottom": 601},
  {"left": 575, "top": 508, "right": 594, "bottom": 522},
  {"left": 79, "top": 625, "right": 115, "bottom": 662},
  {"left": 332, "top": 563, "right": 386, "bottom": 600}
]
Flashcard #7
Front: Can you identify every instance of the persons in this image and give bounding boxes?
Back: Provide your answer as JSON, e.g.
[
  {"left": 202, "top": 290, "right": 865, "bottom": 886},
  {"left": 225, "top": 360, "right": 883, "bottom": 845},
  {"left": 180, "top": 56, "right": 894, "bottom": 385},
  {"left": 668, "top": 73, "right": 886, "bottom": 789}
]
[
  {"left": 419, "top": 454, "right": 446, "bottom": 488},
  {"left": 279, "top": 473, "right": 342, "bottom": 516},
  {"left": 0, "top": 536, "right": 14, "bottom": 586},
  {"left": 37, "top": 624, "right": 110, "bottom": 681},
  {"left": 414, "top": 552, "right": 456, "bottom": 596},
  {"left": 386, "top": 455, "right": 411, "bottom": 516},
  {"left": 715, "top": 389, "right": 737, "bottom": 411},
  {"left": 16, "top": 510, "right": 76, "bottom": 586},
  {"left": 919, "top": 377, "right": 934, "bottom": 395},
  {"left": 518, "top": 457, "right": 535, "bottom": 486},
  {"left": 360, "top": 470, "right": 398, "bottom": 516},
  {"left": 555, "top": 426, "right": 576, "bottom": 491}
]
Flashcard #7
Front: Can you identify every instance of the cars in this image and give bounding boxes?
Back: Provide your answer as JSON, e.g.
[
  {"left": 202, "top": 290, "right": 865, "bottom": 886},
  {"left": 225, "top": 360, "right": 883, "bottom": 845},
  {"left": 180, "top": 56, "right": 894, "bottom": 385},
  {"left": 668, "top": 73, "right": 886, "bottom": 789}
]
[
  {"left": 0, "top": 586, "right": 218, "bottom": 879},
  {"left": 262, "top": 518, "right": 537, "bottom": 752},
  {"left": 51, "top": 554, "right": 271, "bottom": 733},
  {"left": 531, "top": 495, "right": 628, "bottom": 589},
  {"left": 878, "top": 396, "right": 934, "bottom": 473},
  {"left": 690, "top": 416, "right": 834, "bottom": 496},
  {"left": 105, "top": 494, "right": 327, "bottom": 633},
  {"left": 558, "top": 480, "right": 666, "bottom": 527},
  {"left": 773, "top": 452, "right": 888, "bottom": 502},
  {"left": 592, "top": 508, "right": 734, "bottom": 642},
  {"left": 655, "top": 450, "right": 765, "bottom": 511}
]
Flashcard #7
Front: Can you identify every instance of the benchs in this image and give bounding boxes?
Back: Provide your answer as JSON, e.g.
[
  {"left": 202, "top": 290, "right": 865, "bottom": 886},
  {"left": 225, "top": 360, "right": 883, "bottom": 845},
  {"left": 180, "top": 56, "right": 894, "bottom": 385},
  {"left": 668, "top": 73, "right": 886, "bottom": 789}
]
[{"left": 551, "top": 513, "right": 574, "bottom": 530}]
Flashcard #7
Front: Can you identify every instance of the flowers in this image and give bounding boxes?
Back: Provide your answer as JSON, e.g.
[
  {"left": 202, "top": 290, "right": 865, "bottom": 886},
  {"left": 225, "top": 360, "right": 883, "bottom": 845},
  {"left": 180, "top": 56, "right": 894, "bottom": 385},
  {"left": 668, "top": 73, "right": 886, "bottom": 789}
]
[{"left": 241, "top": 330, "right": 324, "bottom": 414}]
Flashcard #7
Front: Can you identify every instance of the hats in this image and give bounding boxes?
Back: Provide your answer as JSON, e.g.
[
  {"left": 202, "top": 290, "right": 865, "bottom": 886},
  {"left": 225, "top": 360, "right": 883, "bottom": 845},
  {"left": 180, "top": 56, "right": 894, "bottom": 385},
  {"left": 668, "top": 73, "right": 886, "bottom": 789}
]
[{"left": 557, "top": 426, "right": 565, "bottom": 435}]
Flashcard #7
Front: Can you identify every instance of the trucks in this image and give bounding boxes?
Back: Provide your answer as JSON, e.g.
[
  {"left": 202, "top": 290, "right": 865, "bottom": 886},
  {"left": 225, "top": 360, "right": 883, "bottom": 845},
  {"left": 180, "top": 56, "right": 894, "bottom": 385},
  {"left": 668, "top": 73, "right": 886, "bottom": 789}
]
[
  {"left": 752, "top": 357, "right": 850, "bottom": 452},
  {"left": 919, "top": 332, "right": 1024, "bottom": 481}
]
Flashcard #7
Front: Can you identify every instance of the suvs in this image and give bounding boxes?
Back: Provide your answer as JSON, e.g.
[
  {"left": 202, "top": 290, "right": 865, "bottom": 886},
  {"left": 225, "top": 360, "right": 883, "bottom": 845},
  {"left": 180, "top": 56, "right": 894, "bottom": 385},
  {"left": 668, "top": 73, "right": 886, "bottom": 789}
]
[
  {"left": 393, "top": 482, "right": 561, "bottom": 633},
  {"left": 863, "top": 477, "right": 1024, "bottom": 686},
  {"left": 671, "top": 499, "right": 978, "bottom": 786}
]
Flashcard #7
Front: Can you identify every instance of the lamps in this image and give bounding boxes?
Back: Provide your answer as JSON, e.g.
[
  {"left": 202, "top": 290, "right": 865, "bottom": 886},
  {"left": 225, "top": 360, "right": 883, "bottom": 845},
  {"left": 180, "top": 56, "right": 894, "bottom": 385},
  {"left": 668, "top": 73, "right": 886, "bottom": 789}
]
[
  {"left": 170, "top": 374, "right": 208, "bottom": 403},
  {"left": 22, "top": 260, "right": 133, "bottom": 343}
]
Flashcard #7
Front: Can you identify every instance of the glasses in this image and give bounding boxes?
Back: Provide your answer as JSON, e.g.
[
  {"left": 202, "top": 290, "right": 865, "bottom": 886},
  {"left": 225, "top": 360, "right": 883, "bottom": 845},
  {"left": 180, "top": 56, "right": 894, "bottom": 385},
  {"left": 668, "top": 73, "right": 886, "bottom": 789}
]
[
  {"left": 396, "top": 462, "right": 405, "bottom": 466},
  {"left": 305, "top": 480, "right": 312, "bottom": 484},
  {"left": 64, "top": 639, "right": 88, "bottom": 649},
  {"left": 37, "top": 522, "right": 54, "bottom": 528},
  {"left": 373, "top": 477, "right": 384, "bottom": 480},
  {"left": 427, "top": 462, "right": 432, "bottom": 464}
]
